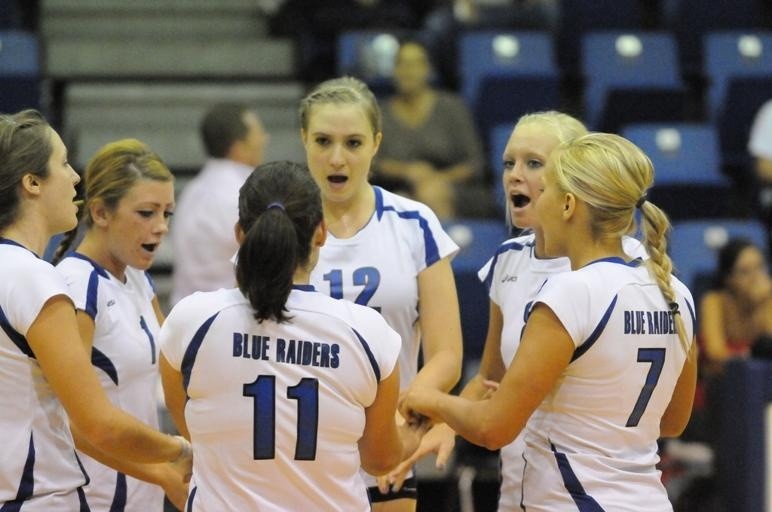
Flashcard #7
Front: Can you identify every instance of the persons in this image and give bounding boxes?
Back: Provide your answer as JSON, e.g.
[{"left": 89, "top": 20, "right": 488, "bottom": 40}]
[
  {"left": 377, "top": 110, "right": 593, "bottom": 512},
  {"left": 395, "top": 132, "right": 697, "bottom": 512},
  {"left": 747, "top": 97, "right": 772, "bottom": 211},
  {"left": 158, "top": 161, "right": 434, "bottom": 512},
  {"left": 51, "top": 137, "right": 190, "bottom": 512},
  {"left": 694, "top": 237, "right": 771, "bottom": 413},
  {"left": 370, "top": 43, "right": 489, "bottom": 221},
  {"left": 0, "top": 108, "right": 193, "bottom": 512},
  {"left": 170, "top": 101, "right": 270, "bottom": 310},
  {"left": 230, "top": 77, "right": 463, "bottom": 512}
]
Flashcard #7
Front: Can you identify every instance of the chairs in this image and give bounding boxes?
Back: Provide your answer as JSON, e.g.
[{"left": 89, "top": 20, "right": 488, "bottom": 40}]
[
  {"left": 338, "top": 32, "right": 401, "bottom": 94},
  {"left": 441, "top": 218, "right": 505, "bottom": 271},
  {"left": 624, "top": 120, "right": 719, "bottom": 184},
  {"left": 700, "top": 31, "right": 772, "bottom": 111},
  {"left": 459, "top": 30, "right": 555, "bottom": 114},
  {"left": 491, "top": 123, "right": 513, "bottom": 208},
  {"left": 581, "top": 30, "right": 681, "bottom": 115},
  {"left": 667, "top": 218, "right": 764, "bottom": 283}
]
